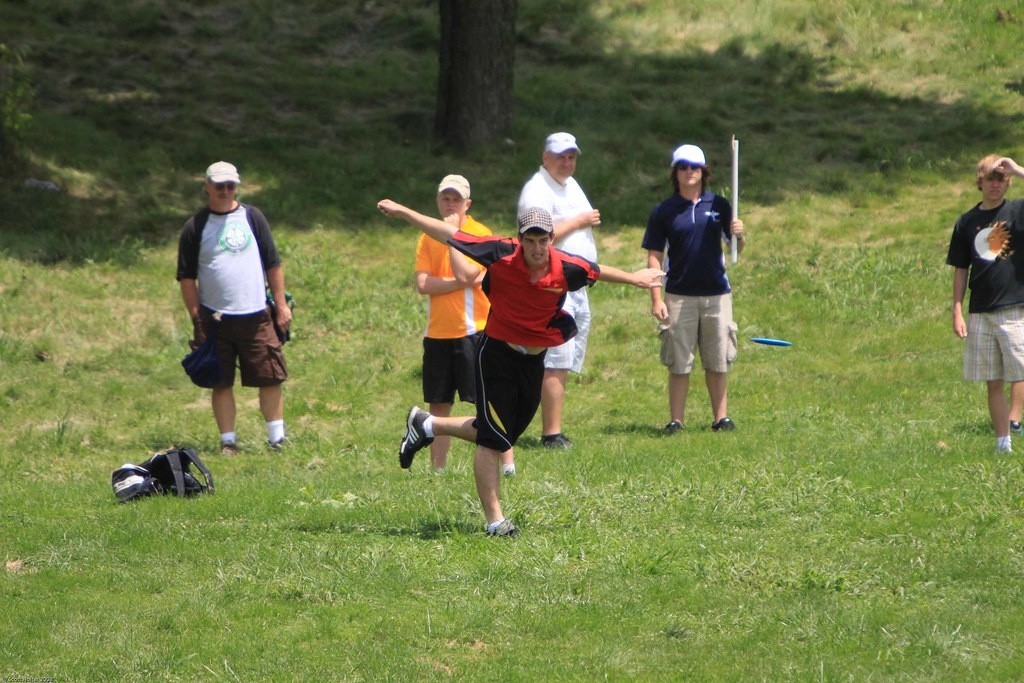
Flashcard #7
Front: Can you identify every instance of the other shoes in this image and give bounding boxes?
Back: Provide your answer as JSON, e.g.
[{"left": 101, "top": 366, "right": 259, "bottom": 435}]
[
  {"left": 711, "top": 417, "right": 735, "bottom": 430},
  {"left": 220, "top": 442, "right": 237, "bottom": 455},
  {"left": 269, "top": 439, "right": 284, "bottom": 452},
  {"left": 1009, "top": 420, "right": 1021, "bottom": 435},
  {"left": 665, "top": 420, "right": 681, "bottom": 434},
  {"left": 540, "top": 435, "right": 570, "bottom": 448}
]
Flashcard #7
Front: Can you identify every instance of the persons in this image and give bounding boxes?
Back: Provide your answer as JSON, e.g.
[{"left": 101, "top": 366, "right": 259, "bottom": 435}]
[
  {"left": 518, "top": 132, "right": 601, "bottom": 449},
  {"left": 176, "top": 160, "right": 292, "bottom": 459},
  {"left": 416, "top": 174, "right": 516, "bottom": 480},
  {"left": 640, "top": 144, "right": 745, "bottom": 436},
  {"left": 946, "top": 153, "right": 1024, "bottom": 458},
  {"left": 377, "top": 199, "right": 667, "bottom": 541}
]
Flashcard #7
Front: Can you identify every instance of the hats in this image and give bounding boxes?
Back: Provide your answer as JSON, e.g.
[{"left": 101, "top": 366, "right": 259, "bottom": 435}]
[
  {"left": 670, "top": 144, "right": 705, "bottom": 168},
  {"left": 976, "top": 153, "right": 1013, "bottom": 182},
  {"left": 206, "top": 160, "right": 241, "bottom": 184},
  {"left": 438, "top": 174, "right": 470, "bottom": 199},
  {"left": 517, "top": 208, "right": 554, "bottom": 234},
  {"left": 545, "top": 132, "right": 581, "bottom": 154}
]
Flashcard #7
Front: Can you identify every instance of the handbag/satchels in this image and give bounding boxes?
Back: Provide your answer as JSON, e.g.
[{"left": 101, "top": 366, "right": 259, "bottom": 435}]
[{"left": 182, "top": 331, "right": 220, "bottom": 387}]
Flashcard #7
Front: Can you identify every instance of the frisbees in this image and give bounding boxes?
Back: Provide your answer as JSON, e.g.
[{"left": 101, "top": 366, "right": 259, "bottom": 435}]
[{"left": 750, "top": 338, "right": 791, "bottom": 346}]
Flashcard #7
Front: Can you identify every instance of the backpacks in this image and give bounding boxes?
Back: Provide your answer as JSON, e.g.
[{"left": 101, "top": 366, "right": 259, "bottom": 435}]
[{"left": 111, "top": 448, "right": 214, "bottom": 504}]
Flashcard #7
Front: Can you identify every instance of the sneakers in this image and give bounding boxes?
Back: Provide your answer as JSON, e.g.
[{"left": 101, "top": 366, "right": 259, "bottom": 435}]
[
  {"left": 489, "top": 522, "right": 519, "bottom": 539},
  {"left": 400, "top": 406, "right": 435, "bottom": 469}
]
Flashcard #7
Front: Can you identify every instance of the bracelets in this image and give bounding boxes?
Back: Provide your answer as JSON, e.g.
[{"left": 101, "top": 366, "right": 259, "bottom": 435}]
[{"left": 737, "top": 235, "right": 743, "bottom": 242}]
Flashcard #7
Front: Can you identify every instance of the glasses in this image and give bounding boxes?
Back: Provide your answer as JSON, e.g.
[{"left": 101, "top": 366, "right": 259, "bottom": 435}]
[
  {"left": 211, "top": 182, "right": 238, "bottom": 191},
  {"left": 676, "top": 165, "right": 700, "bottom": 171}
]
